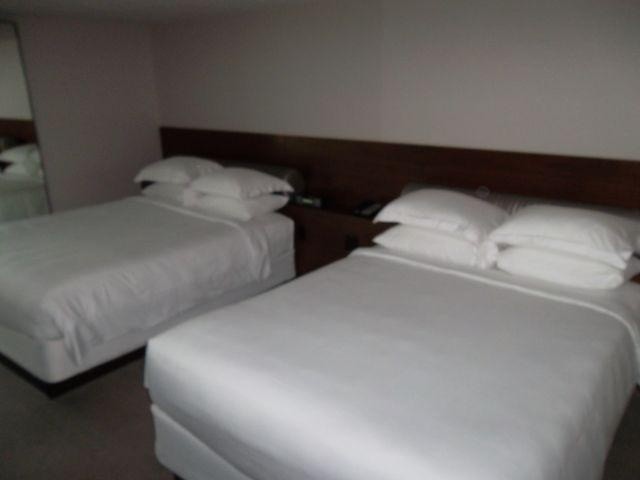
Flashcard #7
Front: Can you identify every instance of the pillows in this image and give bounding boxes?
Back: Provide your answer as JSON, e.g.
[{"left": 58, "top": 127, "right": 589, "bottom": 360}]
[
  {"left": 372, "top": 185, "right": 505, "bottom": 245},
  {"left": 189, "top": 192, "right": 288, "bottom": 220},
  {"left": 186, "top": 168, "right": 293, "bottom": 201},
  {"left": 3, "top": 144, "right": 39, "bottom": 166},
  {"left": 143, "top": 180, "right": 189, "bottom": 205},
  {"left": 5, "top": 165, "right": 48, "bottom": 183},
  {"left": 497, "top": 243, "right": 639, "bottom": 292},
  {"left": 372, "top": 224, "right": 502, "bottom": 272},
  {"left": 134, "top": 156, "right": 221, "bottom": 187},
  {"left": 487, "top": 205, "right": 640, "bottom": 271}
]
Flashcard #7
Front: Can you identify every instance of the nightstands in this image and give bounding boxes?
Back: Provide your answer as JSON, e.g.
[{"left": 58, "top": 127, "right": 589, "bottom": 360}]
[{"left": 297, "top": 202, "right": 392, "bottom": 279}]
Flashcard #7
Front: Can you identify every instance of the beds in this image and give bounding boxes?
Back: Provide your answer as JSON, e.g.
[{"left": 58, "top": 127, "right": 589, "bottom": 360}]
[
  {"left": 0, "top": 163, "right": 317, "bottom": 402},
  {"left": 1, "top": 170, "right": 51, "bottom": 223},
  {"left": 144, "top": 183, "right": 637, "bottom": 478}
]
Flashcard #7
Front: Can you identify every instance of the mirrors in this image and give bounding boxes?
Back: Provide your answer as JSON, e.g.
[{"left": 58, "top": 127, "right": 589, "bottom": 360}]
[{"left": 1, "top": 21, "right": 53, "bottom": 226}]
